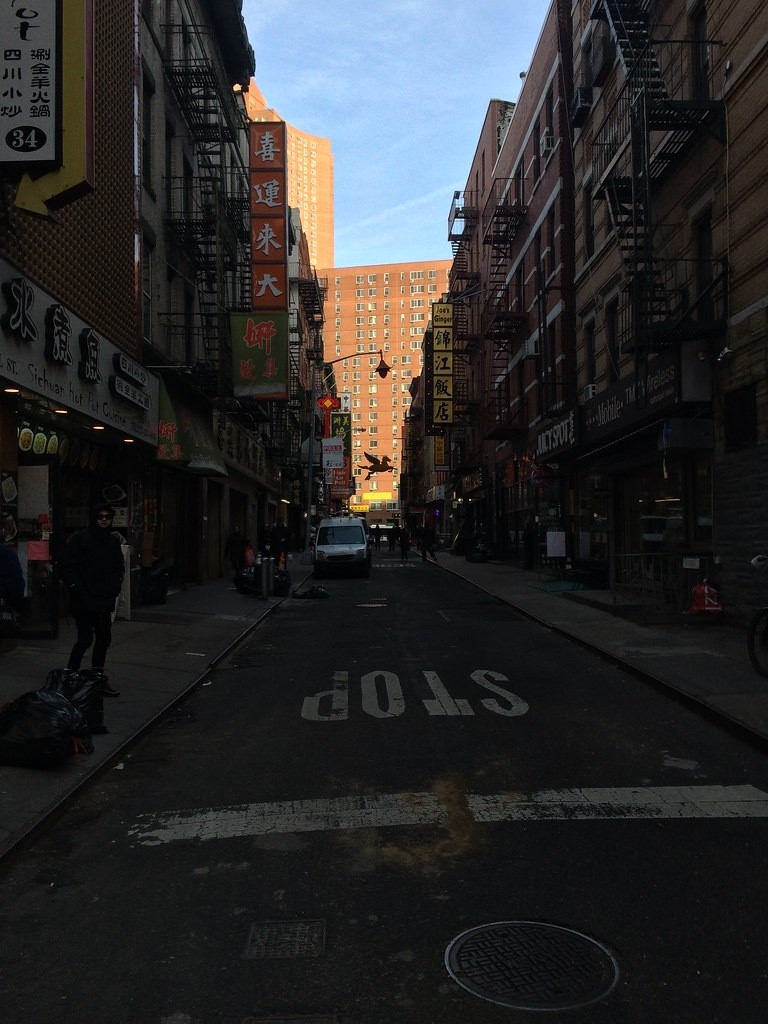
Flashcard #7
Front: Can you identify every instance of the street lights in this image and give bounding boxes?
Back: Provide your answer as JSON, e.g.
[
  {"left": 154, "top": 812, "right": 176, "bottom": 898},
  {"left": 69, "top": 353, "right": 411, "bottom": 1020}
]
[
  {"left": 321, "top": 428, "right": 367, "bottom": 518},
  {"left": 304, "top": 348, "right": 393, "bottom": 552}
]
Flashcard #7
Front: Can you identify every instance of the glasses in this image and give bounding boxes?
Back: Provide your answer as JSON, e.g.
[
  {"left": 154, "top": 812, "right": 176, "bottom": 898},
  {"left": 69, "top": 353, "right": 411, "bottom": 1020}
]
[{"left": 95, "top": 513, "right": 113, "bottom": 520}]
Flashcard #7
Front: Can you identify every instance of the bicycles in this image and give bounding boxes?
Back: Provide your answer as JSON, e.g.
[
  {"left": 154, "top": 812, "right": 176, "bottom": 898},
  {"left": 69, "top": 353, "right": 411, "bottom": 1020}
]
[{"left": 745, "top": 554, "right": 768, "bottom": 679}]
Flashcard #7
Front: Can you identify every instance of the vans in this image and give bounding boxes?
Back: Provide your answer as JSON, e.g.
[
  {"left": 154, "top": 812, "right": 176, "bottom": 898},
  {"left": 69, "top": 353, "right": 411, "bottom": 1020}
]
[{"left": 308, "top": 515, "right": 372, "bottom": 578}]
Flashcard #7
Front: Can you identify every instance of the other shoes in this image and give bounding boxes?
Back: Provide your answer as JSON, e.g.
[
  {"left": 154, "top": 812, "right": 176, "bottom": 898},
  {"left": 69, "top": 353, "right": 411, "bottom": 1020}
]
[{"left": 101, "top": 683, "right": 120, "bottom": 697}]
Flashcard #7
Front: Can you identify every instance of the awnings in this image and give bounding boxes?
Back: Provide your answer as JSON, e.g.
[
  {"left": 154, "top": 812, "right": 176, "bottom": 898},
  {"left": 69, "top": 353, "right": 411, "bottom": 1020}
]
[{"left": 150, "top": 371, "right": 229, "bottom": 478}]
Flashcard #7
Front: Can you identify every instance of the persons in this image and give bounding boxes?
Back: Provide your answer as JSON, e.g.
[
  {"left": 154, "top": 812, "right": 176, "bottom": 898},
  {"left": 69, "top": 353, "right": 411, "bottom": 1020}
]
[
  {"left": 521, "top": 523, "right": 535, "bottom": 570},
  {"left": 398, "top": 529, "right": 409, "bottom": 561},
  {"left": 420, "top": 523, "right": 437, "bottom": 563},
  {"left": 373, "top": 526, "right": 381, "bottom": 553},
  {"left": 223, "top": 523, "right": 251, "bottom": 577},
  {"left": 60, "top": 503, "right": 121, "bottom": 698},
  {"left": 387, "top": 528, "right": 395, "bottom": 552}
]
